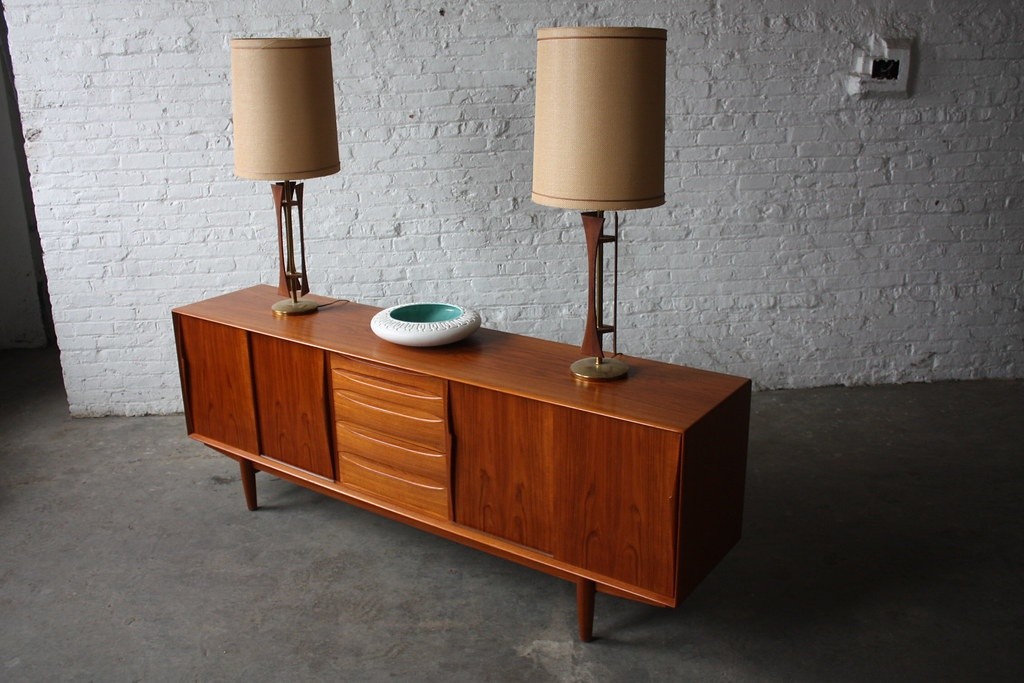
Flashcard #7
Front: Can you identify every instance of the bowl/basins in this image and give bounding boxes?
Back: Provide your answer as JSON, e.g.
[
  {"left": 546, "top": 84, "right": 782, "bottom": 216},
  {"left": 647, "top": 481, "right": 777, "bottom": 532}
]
[{"left": 370, "top": 302, "right": 481, "bottom": 346}]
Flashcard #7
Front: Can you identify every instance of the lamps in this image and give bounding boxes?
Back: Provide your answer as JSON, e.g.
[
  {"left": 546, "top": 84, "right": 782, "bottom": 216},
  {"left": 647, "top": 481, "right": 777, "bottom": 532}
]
[
  {"left": 231, "top": 37, "right": 341, "bottom": 316},
  {"left": 530, "top": 27, "right": 669, "bottom": 380}
]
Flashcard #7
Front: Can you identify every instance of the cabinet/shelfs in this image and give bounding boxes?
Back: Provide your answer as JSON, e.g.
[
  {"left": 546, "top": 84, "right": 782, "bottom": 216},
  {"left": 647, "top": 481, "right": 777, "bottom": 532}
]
[{"left": 170, "top": 285, "right": 752, "bottom": 643}]
